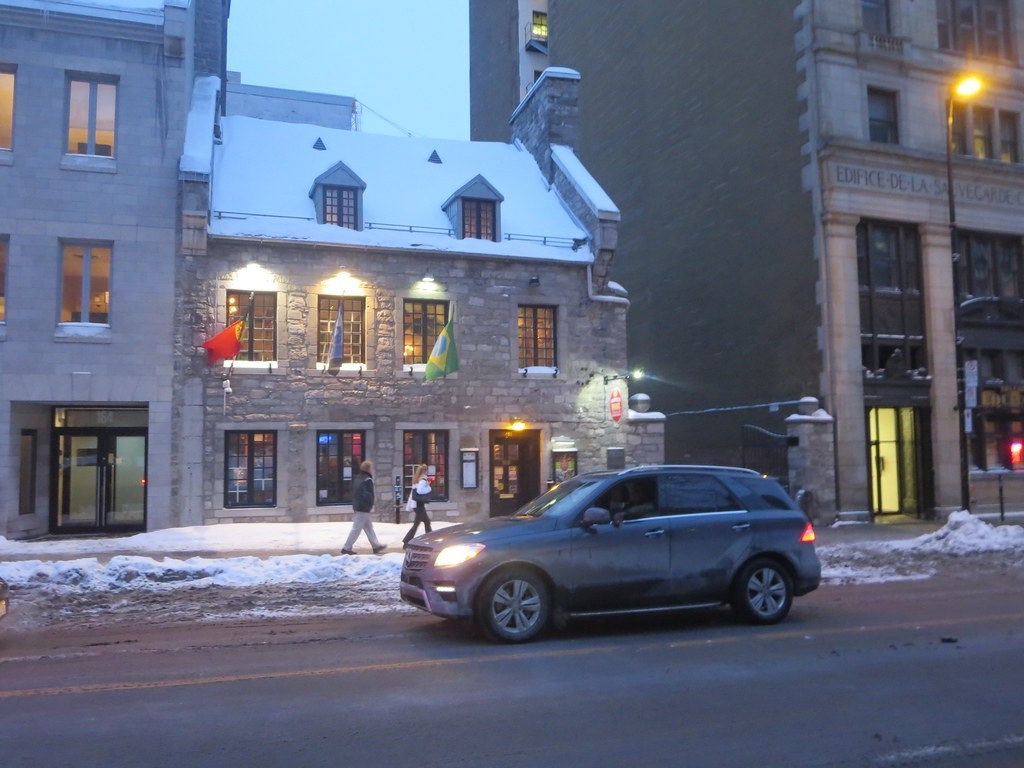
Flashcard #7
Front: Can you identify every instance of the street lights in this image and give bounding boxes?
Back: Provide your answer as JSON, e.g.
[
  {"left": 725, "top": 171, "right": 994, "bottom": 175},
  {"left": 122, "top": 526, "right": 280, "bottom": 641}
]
[{"left": 945, "top": 74, "right": 984, "bottom": 526}]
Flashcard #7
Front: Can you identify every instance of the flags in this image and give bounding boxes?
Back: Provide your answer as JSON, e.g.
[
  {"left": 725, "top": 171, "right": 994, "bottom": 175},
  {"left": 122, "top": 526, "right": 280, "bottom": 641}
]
[
  {"left": 424, "top": 319, "right": 460, "bottom": 379},
  {"left": 327, "top": 303, "right": 346, "bottom": 373},
  {"left": 203, "top": 317, "right": 250, "bottom": 363}
]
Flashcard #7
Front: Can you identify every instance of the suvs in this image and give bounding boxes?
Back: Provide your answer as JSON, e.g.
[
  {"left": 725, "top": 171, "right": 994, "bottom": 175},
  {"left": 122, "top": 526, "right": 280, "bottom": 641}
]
[{"left": 399, "top": 464, "right": 823, "bottom": 642}]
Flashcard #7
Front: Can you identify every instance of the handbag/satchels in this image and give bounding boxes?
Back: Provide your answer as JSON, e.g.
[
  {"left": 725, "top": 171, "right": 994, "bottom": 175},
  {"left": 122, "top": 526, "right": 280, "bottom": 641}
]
[{"left": 412, "top": 479, "right": 431, "bottom": 503}]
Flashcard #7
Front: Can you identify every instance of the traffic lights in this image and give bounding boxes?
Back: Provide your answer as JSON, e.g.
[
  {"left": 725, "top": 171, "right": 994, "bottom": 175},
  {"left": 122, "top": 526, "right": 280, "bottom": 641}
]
[{"left": 1002, "top": 437, "right": 1024, "bottom": 470}]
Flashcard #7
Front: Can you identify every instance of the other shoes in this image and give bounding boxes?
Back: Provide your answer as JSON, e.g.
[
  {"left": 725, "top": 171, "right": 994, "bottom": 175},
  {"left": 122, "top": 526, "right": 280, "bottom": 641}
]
[
  {"left": 372, "top": 545, "right": 388, "bottom": 554},
  {"left": 341, "top": 549, "right": 356, "bottom": 555}
]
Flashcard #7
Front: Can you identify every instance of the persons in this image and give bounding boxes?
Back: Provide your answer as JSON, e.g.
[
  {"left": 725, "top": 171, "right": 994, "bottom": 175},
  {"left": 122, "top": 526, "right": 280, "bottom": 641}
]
[
  {"left": 402, "top": 464, "right": 434, "bottom": 550},
  {"left": 342, "top": 461, "right": 388, "bottom": 555}
]
